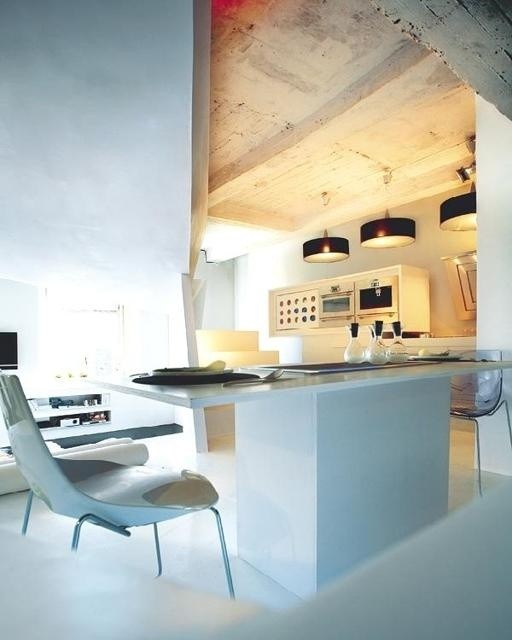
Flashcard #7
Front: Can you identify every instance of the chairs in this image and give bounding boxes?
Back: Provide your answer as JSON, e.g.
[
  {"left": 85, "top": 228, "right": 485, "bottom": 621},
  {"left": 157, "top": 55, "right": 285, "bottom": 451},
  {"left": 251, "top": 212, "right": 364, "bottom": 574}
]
[
  {"left": 443, "top": 347, "right": 512, "bottom": 498},
  {"left": 1, "top": 372, "right": 241, "bottom": 606}
]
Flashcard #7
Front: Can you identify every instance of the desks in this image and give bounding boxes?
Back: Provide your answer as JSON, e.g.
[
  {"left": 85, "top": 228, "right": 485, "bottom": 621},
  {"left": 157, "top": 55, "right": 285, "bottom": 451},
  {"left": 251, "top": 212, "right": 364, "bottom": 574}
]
[{"left": 90, "top": 359, "right": 511, "bottom": 607}]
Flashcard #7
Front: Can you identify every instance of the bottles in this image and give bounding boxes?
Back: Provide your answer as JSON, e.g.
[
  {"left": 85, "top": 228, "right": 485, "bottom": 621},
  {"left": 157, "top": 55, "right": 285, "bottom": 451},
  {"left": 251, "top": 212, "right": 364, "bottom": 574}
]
[
  {"left": 366, "top": 318, "right": 392, "bottom": 365},
  {"left": 389, "top": 320, "right": 410, "bottom": 364},
  {"left": 342, "top": 321, "right": 366, "bottom": 365}
]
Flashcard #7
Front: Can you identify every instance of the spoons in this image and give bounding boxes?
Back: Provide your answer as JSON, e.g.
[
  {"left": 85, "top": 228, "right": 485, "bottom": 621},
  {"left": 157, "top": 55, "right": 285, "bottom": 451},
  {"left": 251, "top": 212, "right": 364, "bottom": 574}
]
[{"left": 220, "top": 366, "right": 285, "bottom": 388}]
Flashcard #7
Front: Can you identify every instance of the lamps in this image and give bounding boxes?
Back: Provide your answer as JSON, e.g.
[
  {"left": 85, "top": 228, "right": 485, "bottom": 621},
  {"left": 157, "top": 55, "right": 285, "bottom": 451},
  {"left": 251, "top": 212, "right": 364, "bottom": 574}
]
[{"left": 299, "top": 187, "right": 478, "bottom": 264}]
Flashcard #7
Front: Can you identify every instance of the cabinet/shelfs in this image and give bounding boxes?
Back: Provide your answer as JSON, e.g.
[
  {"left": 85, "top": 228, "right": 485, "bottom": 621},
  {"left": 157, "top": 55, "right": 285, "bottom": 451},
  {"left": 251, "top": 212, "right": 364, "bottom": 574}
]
[{"left": 263, "top": 264, "right": 430, "bottom": 337}]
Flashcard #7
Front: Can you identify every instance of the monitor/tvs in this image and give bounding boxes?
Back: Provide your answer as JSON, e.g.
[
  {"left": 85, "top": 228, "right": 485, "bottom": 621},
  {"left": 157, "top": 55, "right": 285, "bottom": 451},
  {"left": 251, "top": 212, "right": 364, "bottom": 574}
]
[{"left": 0, "top": 331, "right": 19, "bottom": 369}]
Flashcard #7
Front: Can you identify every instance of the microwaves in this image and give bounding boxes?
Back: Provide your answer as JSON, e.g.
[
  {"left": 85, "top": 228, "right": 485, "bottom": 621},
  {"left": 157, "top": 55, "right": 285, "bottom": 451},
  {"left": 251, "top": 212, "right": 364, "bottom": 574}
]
[
  {"left": 319, "top": 290, "right": 355, "bottom": 319},
  {"left": 355, "top": 275, "right": 398, "bottom": 316}
]
